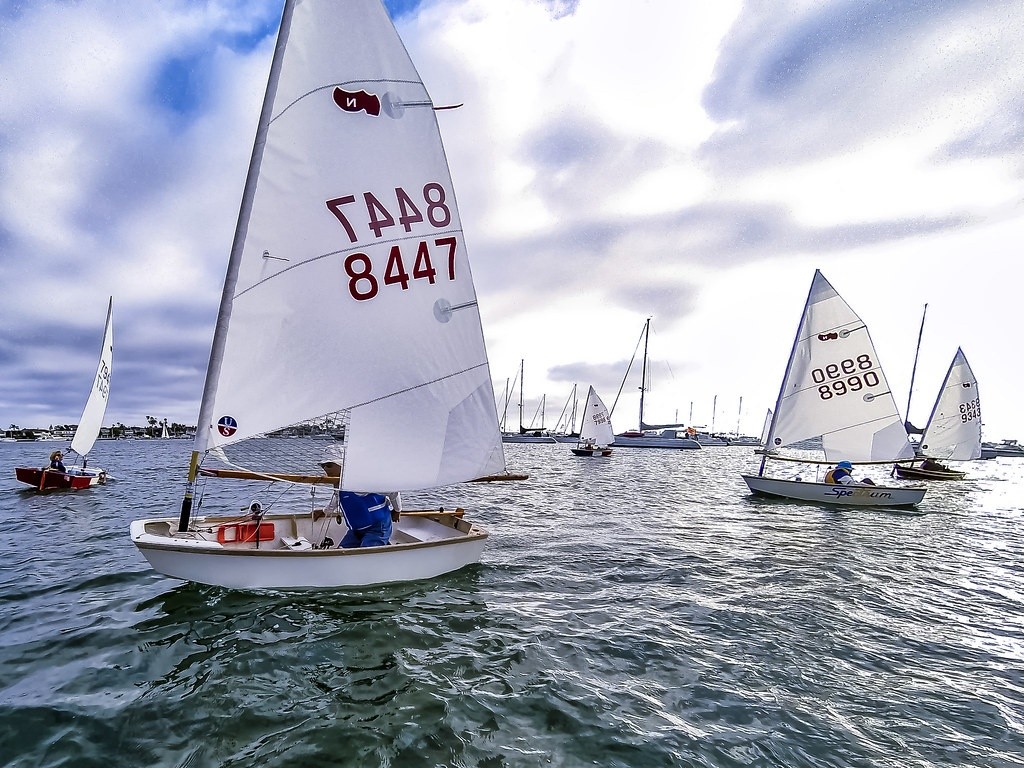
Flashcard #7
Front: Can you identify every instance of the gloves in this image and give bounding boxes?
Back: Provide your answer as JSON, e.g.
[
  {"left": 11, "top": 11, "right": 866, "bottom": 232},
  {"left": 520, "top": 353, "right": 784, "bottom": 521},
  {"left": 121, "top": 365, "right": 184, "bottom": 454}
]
[
  {"left": 311, "top": 510, "right": 326, "bottom": 522},
  {"left": 391, "top": 510, "right": 400, "bottom": 522}
]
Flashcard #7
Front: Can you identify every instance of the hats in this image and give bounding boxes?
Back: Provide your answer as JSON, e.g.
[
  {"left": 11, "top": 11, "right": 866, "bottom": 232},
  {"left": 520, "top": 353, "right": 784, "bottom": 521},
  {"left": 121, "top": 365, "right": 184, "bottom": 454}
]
[
  {"left": 318, "top": 445, "right": 343, "bottom": 468},
  {"left": 836, "top": 461, "right": 855, "bottom": 470}
]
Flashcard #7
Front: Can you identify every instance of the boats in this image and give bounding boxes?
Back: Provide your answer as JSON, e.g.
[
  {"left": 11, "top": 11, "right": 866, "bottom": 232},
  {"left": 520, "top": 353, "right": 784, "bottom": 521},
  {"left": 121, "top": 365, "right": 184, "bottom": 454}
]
[
  {"left": 994, "top": 439, "right": 1024, "bottom": 458},
  {"left": 981, "top": 442, "right": 997, "bottom": 460},
  {"left": 0, "top": 434, "right": 74, "bottom": 442}
]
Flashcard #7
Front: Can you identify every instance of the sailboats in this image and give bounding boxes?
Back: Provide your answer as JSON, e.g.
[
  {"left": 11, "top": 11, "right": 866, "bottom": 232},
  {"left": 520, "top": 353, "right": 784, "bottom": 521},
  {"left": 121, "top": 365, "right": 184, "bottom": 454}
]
[
  {"left": 897, "top": 346, "right": 982, "bottom": 480},
  {"left": 161, "top": 423, "right": 172, "bottom": 440},
  {"left": 739, "top": 270, "right": 928, "bottom": 509},
  {"left": 905, "top": 302, "right": 929, "bottom": 448},
  {"left": 570, "top": 385, "right": 616, "bottom": 458},
  {"left": 605, "top": 317, "right": 772, "bottom": 451},
  {"left": 128, "top": 0, "right": 531, "bottom": 592},
  {"left": 13, "top": 295, "right": 117, "bottom": 491},
  {"left": 496, "top": 359, "right": 578, "bottom": 442}
]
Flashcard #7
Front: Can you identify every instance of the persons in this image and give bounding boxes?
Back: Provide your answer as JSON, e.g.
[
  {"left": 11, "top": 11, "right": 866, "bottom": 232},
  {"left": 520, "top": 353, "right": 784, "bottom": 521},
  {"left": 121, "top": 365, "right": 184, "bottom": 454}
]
[
  {"left": 310, "top": 445, "right": 402, "bottom": 549},
  {"left": 50, "top": 451, "right": 66, "bottom": 472},
  {"left": 832, "top": 461, "right": 875, "bottom": 486}
]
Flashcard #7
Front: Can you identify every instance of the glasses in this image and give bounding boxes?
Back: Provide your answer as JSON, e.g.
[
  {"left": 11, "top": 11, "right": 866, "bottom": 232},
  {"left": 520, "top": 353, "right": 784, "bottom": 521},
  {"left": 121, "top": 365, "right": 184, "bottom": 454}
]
[{"left": 321, "top": 462, "right": 336, "bottom": 467}]
